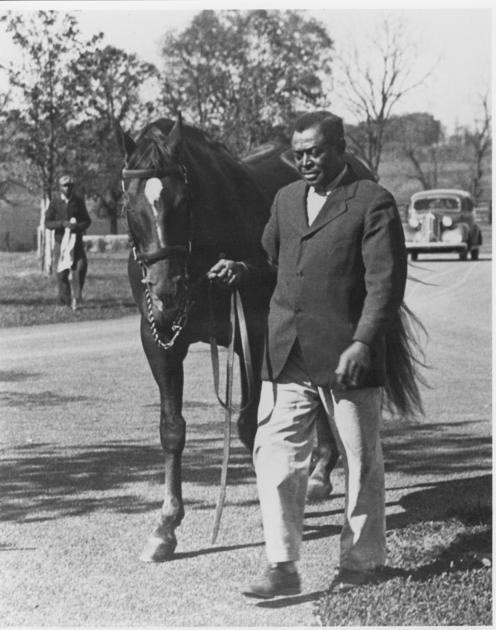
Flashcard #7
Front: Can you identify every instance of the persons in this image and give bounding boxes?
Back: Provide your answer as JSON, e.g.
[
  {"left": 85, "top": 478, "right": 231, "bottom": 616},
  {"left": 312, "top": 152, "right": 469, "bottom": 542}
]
[
  {"left": 206, "top": 111, "right": 407, "bottom": 599},
  {"left": 43, "top": 176, "right": 91, "bottom": 313}
]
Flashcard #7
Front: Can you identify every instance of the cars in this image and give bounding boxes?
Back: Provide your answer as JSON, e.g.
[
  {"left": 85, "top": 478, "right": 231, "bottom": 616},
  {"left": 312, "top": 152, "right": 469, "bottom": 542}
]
[{"left": 402, "top": 188, "right": 483, "bottom": 261}]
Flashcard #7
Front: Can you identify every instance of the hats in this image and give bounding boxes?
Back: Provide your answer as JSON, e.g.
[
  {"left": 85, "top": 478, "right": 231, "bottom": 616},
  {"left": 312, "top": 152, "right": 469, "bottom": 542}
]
[{"left": 58, "top": 175, "right": 78, "bottom": 184}]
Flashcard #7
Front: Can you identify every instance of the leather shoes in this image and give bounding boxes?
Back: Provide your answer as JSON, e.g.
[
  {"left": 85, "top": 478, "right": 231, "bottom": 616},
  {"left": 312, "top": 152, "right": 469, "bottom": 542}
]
[
  {"left": 332, "top": 564, "right": 389, "bottom": 593},
  {"left": 243, "top": 566, "right": 301, "bottom": 601}
]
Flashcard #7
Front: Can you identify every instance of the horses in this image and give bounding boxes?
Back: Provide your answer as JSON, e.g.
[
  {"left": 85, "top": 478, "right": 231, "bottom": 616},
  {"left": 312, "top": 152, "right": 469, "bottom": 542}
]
[{"left": 112, "top": 109, "right": 440, "bottom": 565}]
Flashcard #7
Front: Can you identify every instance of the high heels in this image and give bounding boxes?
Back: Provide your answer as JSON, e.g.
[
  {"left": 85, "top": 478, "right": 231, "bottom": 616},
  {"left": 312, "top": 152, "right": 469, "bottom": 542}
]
[{"left": 60, "top": 297, "right": 87, "bottom": 311}]
